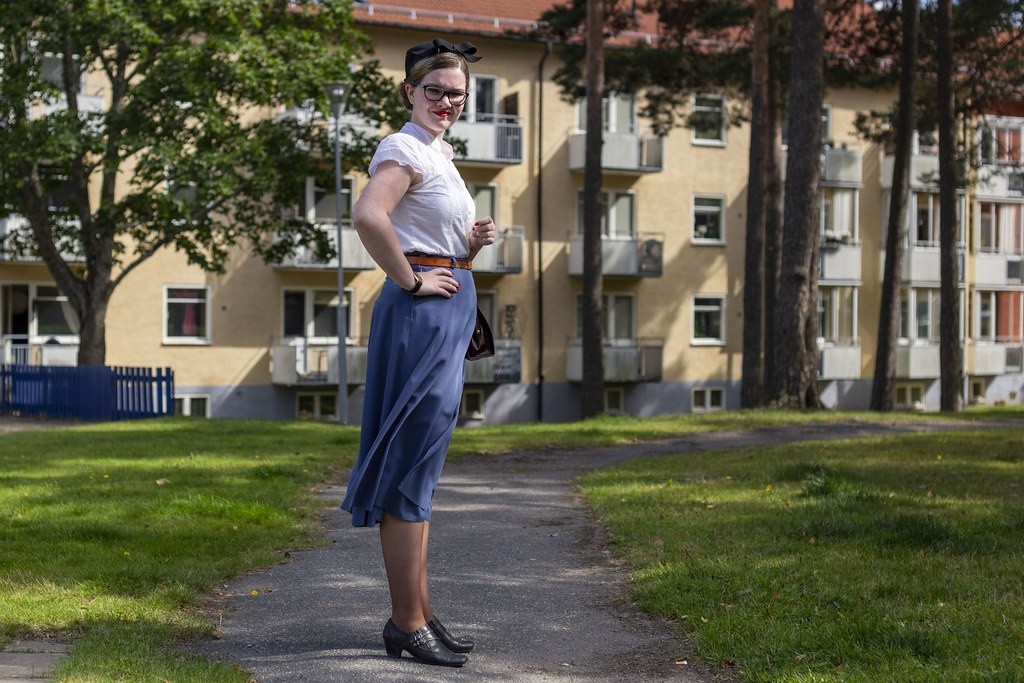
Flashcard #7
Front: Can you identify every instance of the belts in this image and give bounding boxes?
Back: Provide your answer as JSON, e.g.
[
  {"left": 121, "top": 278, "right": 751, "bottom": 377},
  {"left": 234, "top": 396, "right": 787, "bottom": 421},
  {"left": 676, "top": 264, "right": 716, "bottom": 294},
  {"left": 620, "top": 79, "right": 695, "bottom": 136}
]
[{"left": 406, "top": 256, "right": 473, "bottom": 270}]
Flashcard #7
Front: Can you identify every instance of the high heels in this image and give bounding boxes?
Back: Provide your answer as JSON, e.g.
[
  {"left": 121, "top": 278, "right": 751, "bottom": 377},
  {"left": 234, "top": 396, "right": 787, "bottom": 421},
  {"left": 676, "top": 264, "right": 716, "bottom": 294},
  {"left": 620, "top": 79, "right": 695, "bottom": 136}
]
[
  {"left": 427, "top": 615, "right": 474, "bottom": 653},
  {"left": 383, "top": 617, "right": 468, "bottom": 666}
]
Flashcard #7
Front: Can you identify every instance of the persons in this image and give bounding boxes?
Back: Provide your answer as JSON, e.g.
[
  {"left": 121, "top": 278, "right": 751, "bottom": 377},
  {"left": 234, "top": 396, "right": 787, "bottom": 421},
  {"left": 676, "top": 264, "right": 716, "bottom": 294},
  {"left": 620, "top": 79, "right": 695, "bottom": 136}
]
[{"left": 341, "top": 39, "right": 497, "bottom": 665}]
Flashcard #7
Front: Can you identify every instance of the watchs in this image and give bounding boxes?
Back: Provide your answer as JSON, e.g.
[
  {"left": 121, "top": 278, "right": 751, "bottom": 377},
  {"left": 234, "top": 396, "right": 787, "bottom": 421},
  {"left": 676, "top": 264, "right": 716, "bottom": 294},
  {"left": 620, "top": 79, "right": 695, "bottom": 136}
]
[{"left": 400, "top": 273, "right": 422, "bottom": 294}]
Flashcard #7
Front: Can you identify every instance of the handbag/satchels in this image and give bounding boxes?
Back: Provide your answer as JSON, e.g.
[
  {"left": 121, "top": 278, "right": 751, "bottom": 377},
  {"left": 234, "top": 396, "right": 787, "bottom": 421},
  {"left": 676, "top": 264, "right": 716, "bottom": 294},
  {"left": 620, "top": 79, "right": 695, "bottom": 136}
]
[{"left": 465, "top": 305, "right": 495, "bottom": 360}]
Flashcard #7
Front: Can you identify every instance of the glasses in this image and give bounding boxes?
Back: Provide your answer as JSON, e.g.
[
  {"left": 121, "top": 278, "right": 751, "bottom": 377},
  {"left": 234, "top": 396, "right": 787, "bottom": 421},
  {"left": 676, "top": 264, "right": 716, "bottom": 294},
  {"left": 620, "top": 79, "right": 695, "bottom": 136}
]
[{"left": 416, "top": 84, "right": 469, "bottom": 106}]
